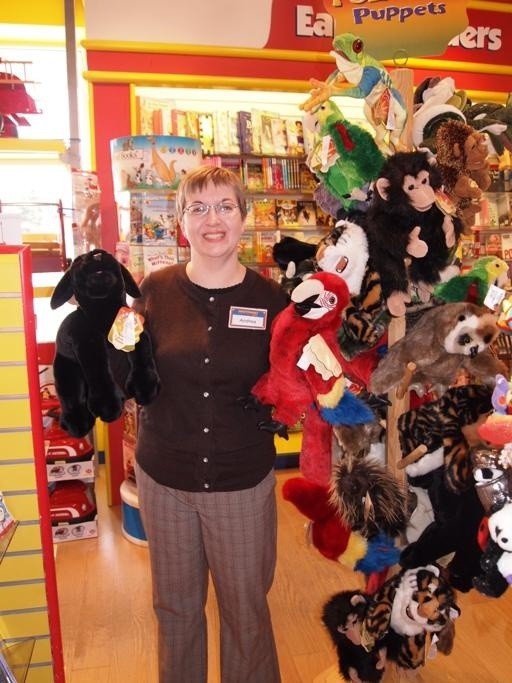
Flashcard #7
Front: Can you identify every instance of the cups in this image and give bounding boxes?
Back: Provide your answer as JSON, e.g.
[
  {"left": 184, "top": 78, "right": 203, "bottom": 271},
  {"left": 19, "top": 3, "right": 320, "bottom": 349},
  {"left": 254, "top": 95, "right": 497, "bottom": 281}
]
[{"left": 183, "top": 204, "right": 241, "bottom": 217}]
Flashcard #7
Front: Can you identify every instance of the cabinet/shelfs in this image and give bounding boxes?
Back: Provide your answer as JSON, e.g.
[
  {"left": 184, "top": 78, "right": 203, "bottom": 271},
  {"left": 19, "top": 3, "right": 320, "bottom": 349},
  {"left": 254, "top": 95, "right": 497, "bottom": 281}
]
[{"left": 1, "top": 135, "right": 512, "bottom": 683}]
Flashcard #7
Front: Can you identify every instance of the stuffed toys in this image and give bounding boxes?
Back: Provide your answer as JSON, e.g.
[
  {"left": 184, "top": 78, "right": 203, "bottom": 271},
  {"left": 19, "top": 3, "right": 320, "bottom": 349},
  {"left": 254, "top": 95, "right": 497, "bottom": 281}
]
[
  {"left": 251, "top": 75, "right": 512, "bottom": 597},
  {"left": 321, "top": 562, "right": 460, "bottom": 683},
  {"left": 300, "top": 33, "right": 408, "bottom": 156}
]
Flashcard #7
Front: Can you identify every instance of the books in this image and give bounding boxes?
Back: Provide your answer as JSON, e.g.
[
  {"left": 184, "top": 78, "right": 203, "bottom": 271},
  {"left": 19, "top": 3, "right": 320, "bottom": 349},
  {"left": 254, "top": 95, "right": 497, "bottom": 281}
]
[
  {"left": 455, "top": 162, "right": 510, "bottom": 280},
  {"left": 135, "top": 90, "right": 335, "bottom": 290}
]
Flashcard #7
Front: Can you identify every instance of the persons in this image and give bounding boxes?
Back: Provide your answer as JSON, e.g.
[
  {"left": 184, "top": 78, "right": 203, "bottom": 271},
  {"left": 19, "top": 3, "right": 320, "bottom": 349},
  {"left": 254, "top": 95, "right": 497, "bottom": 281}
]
[{"left": 109, "top": 167, "right": 290, "bottom": 683}]
